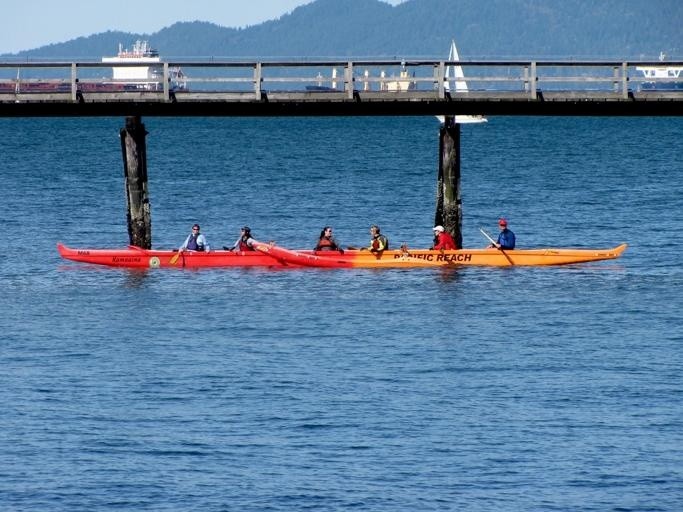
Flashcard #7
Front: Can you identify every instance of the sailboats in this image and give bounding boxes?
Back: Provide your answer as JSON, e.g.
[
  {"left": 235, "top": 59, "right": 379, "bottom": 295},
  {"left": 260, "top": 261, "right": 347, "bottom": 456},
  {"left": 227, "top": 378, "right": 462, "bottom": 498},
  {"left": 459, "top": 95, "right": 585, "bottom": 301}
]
[{"left": 435, "top": 39, "right": 488, "bottom": 124}]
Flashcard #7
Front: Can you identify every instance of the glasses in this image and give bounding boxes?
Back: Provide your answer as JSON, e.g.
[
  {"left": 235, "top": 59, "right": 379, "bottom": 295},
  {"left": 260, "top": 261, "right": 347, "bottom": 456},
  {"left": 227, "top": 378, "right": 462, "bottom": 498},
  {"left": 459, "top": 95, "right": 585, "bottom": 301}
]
[{"left": 192, "top": 228, "right": 199, "bottom": 231}]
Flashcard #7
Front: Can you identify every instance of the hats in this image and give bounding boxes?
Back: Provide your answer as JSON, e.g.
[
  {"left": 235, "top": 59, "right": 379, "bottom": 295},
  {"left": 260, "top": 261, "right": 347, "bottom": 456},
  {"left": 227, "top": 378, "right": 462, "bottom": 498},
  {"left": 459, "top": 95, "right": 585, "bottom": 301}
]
[
  {"left": 432, "top": 225, "right": 445, "bottom": 233},
  {"left": 498, "top": 219, "right": 507, "bottom": 225}
]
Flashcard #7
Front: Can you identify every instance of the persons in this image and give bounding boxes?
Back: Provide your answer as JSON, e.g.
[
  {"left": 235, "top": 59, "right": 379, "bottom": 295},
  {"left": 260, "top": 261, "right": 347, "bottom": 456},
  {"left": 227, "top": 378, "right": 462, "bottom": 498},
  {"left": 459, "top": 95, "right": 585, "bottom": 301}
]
[
  {"left": 433, "top": 225, "right": 457, "bottom": 250},
  {"left": 487, "top": 219, "right": 515, "bottom": 249},
  {"left": 317, "top": 226, "right": 336, "bottom": 251},
  {"left": 178, "top": 224, "right": 211, "bottom": 254},
  {"left": 233, "top": 226, "right": 256, "bottom": 252},
  {"left": 368, "top": 225, "right": 388, "bottom": 252}
]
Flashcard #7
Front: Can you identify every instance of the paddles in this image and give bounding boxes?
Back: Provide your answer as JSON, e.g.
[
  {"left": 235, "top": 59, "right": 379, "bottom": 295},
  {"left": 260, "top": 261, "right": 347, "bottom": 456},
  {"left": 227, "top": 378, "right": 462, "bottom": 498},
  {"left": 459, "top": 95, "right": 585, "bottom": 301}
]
[
  {"left": 223, "top": 246, "right": 237, "bottom": 254},
  {"left": 348, "top": 246, "right": 378, "bottom": 256},
  {"left": 170, "top": 252, "right": 181, "bottom": 264}
]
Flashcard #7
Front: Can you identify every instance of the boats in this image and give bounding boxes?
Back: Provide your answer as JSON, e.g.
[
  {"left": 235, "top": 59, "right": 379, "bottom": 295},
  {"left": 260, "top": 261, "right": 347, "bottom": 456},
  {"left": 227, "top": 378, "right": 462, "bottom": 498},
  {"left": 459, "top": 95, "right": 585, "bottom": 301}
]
[
  {"left": 384, "top": 67, "right": 417, "bottom": 92},
  {"left": 305, "top": 68, "right": 341, "bottom": 91},
  {"left": 56, "top": 242, "right": 315, "bottom": 268},
  {"left": 251, "top": 242, "right": 628, "bottom": 266}
]
